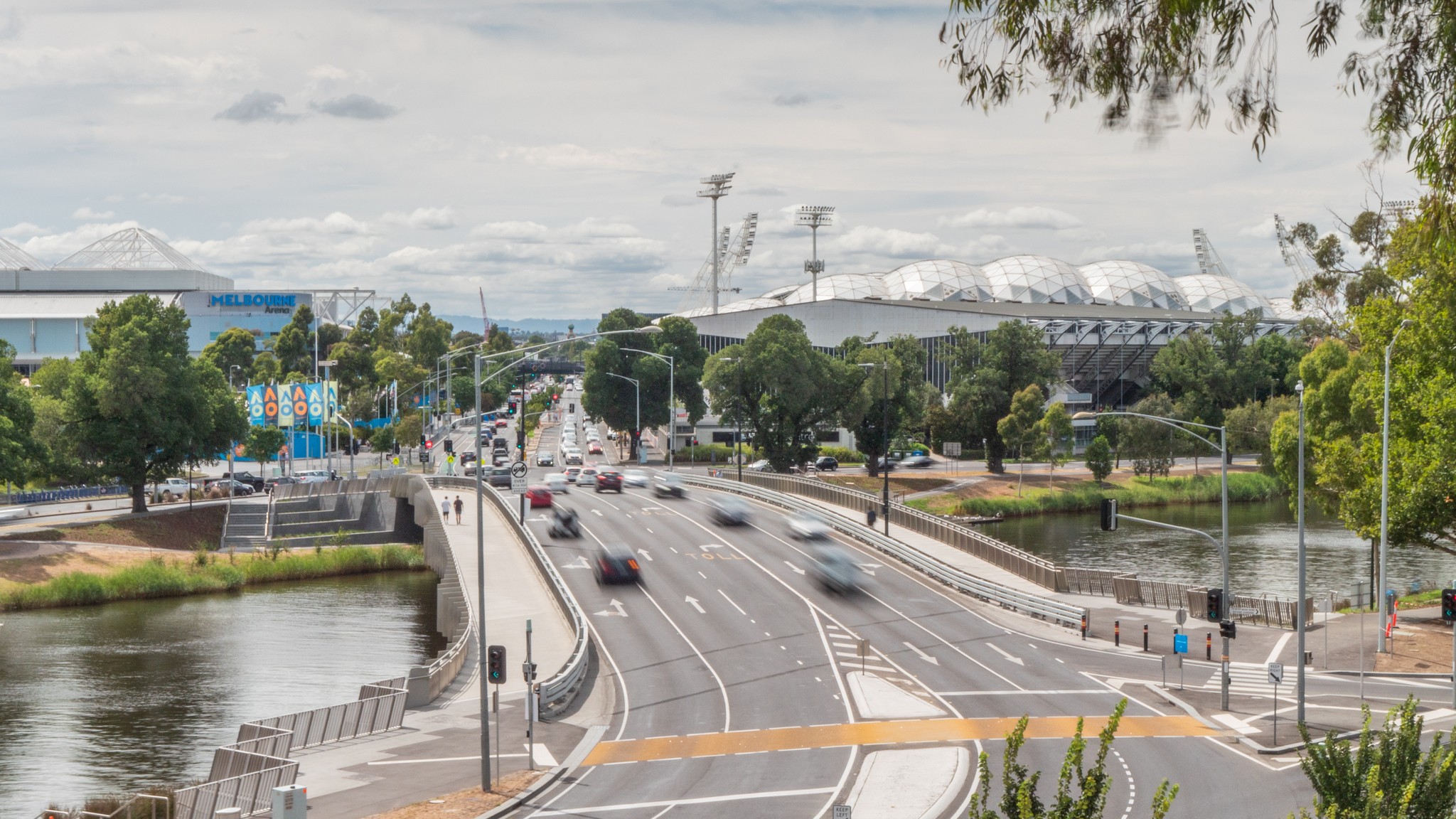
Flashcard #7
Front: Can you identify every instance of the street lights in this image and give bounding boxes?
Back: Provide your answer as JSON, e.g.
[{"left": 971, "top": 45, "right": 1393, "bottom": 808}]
[
  {"left": 1293, "top": 379, "right": 1305, "bottom": 723},
  {"left": 620, "top": 348, "right": 673, "bottom": 472},
  {"left": 1376, "top": 318, "right": 1415, "bottom": 653},
  {"left": 858, "top": 355, "right": 888, "bottom": 538},
  {"left": 446, "top": 340, "right": 489, "bottom": 475},
  {"left": 606, "top": 372, "right": 640, "bottom": 465},
  {"left": 229, "top": 365, "right": 239, "bottom": 499},
  {"left": 1070, "top": 411, "right": 1230, "bottom": 711},
  {"left": 394, "top": 350, "right": 473, "bottom": 475},
  {"left": 477, "top": 324, "right": 664, "bottom": 792},
  {"left": 720, "top": 354, "right": 741, "bottom": 482}
]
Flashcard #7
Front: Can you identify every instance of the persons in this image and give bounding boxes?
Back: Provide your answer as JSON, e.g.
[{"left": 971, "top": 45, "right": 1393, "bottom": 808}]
[
  {"left": 442, "top": 496, "right": 451, "bottom": 524},
  {"left": 453, "top": 495, "right": 464, "bottom": 525},
  {"left": 331, "top": 469, "right": 340, "bottom": 480},
  {"left": 554, "top": 412, "right": 559, "bottom": 422}
]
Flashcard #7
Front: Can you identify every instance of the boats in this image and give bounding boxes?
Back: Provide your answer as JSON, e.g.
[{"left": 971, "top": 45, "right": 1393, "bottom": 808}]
[{"left": 1207, "top": 588, "right": 1223, "bottom": 622}]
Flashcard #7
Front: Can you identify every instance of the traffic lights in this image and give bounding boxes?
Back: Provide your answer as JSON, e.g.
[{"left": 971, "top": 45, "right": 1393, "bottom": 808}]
[
  {"left": 531, "top": 372, "right": 535, "bottom": 379},
  {"left": 546, "top": 400, "right": 550, "bottom": 407},
  {"left": 1442, "top": 588, "right": 1456, "bottom": 621},
  {"left": 512, "top": 383, "right": 515, "bottom": 389},
  {"left": 508, "top": 402, "right": 517, "bottom": 414},
  {"left": 559, "top": 376, "right": 563, "bottom": 382},
  {"left": 553, "top": 394, "right": 559, "bottom": 404},
  {"left": 421, "top": 435, "right": 426, "bottom": 445},
  {"left": 446, "top": 440, "right": 453, "bottom": 452},
  {"left": 636, "top": 431, "right": 641, "bottom": 441},
  {"left": 516, "top": 431, "right": 523, "bottom": 449}
]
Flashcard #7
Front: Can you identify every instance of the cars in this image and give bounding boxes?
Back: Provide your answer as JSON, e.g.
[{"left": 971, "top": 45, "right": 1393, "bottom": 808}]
[
  {"left": 507, "top": 375, "right": 583, "bottom": 406},
  {"left": 460, "top": 412, "right": 876, "bottom": 600}
]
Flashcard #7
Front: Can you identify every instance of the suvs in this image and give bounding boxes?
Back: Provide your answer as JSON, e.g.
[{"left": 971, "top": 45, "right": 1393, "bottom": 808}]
[{"left": 144, "top": 468, "right": 348, "bottom": 504}]
